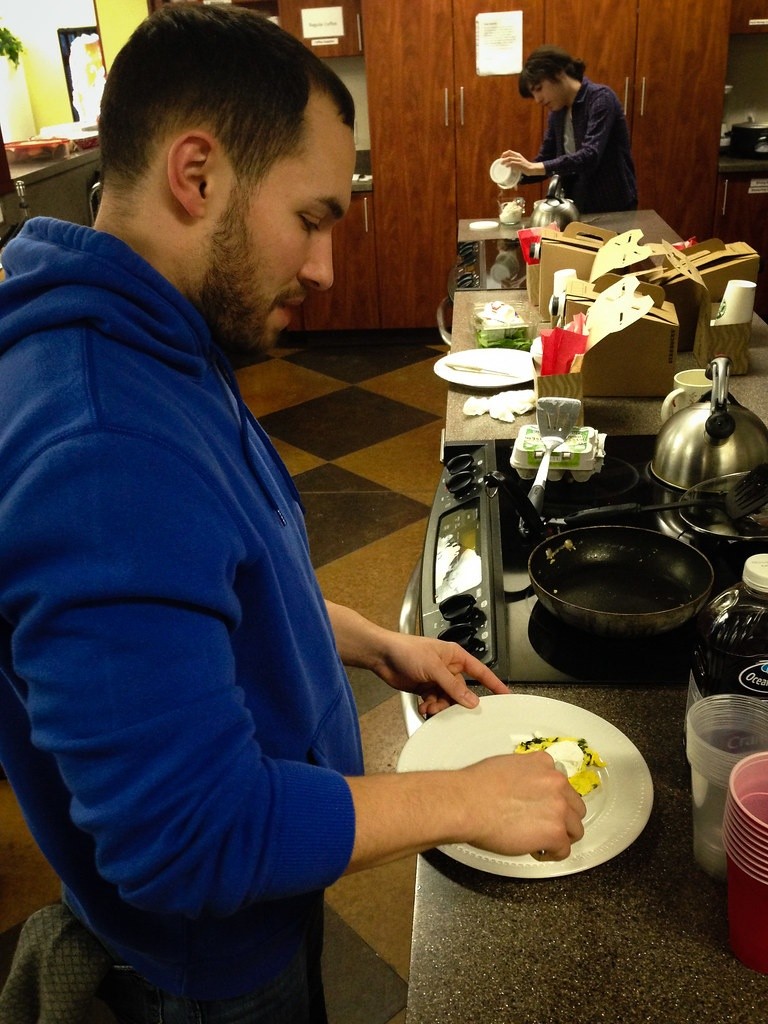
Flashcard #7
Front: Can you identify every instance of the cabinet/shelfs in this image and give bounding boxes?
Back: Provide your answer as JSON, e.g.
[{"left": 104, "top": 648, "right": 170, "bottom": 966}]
[
  {"left": 282, "top": 192, "right": 379, "bottom": 333},
  {"left": 545, "top": 0, "right": 733, "bottom": 243},
  {"left": 362, "top": 0, "right": 545, "bottom": 330},
  {"left": 730, "top": 0, "right": 768, "bottom": 35},
  {"left": 712, "top": 172, "right": 768, "bottom": 319},
  {"left": 145, "top": 0, "right": 364, "bottom": 58}
]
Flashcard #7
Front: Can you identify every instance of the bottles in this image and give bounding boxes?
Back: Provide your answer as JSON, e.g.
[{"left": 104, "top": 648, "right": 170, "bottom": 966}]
[{"left": 681, "top": 554, "right": 768, "bottom": 761}]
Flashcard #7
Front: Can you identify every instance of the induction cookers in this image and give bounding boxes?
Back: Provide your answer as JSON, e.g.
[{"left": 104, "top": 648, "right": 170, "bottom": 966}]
[
  {"left": 454, "top": 235, "right": 528, "bottom": 291},
  {"left": 422, "top": 436, "right": 768, "bottom": 683}
]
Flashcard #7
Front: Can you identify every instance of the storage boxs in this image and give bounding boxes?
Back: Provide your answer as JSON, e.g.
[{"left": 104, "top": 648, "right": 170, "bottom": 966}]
[{"left": 468, "top": 221, "right": 760, "bottom": 426}]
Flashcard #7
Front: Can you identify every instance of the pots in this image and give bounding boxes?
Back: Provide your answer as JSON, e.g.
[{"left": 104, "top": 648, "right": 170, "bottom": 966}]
[
  {"left": 528, "top": 526, "right": 714, "bottom": 634},
  {"left": 724, "top": 117, "right": 768, "bottom": 160},
  {"left": 678, "top": 472, "right": 767, "bottom": 541}
]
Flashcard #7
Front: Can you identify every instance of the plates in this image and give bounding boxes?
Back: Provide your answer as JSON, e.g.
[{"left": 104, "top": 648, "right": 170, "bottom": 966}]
[
  {"left": 397, "top": 694, "right": 654, "bottom": 879},
  {"left": 434, "top": 348, "right": 534, "bottom": 389}
]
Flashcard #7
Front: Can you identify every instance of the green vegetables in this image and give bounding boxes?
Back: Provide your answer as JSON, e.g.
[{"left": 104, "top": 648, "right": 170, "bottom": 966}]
[{"left": 474, "top": 328, "right": 532, "bottom": 351}]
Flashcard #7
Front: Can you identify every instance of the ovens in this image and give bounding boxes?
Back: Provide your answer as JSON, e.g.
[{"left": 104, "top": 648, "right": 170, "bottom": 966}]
[
  {"left": 398, "top": 554, "right": 426, "bottom": 738},
  {"left": 438, "top": 266, "right": 454, "bottom": 348}
]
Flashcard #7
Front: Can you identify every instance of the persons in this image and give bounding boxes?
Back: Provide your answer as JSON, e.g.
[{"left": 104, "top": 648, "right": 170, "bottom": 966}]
[
  {"left": 501, "top": 45, "right": 638, "bottom": 214},
  {"left": 0, "top": 0, "right": 586, "bottom": 1024}
]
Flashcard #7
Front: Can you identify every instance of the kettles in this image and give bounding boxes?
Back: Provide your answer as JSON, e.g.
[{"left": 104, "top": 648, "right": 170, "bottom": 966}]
[
  {"left": 649, "top": 356, "right": 768, "bottom": 495},
  {"left": 524, "top": 175, "right": 580, "bottom": 233}
]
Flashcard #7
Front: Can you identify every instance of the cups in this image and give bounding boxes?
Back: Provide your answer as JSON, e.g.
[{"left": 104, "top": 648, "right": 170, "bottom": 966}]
[
  {"left": 661, "top": 369, "right": 713, "bottom": 424},
  {"left": 686, "top": 694, "right": 768, "bottom": 878},
  {"left": 553, "top": 268, "right": 577, "bottom": 316},
  {"left": 713, "top": 280, "right": 757, "bottom": 327},
  {"left": 722, "top": 752, "right": 768, "bottom": 975}
]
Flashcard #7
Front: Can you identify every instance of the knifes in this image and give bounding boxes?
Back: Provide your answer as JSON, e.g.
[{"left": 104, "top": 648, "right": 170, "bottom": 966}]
[{"left": 446, "top": 363, "right": 516, "bottom": 378}]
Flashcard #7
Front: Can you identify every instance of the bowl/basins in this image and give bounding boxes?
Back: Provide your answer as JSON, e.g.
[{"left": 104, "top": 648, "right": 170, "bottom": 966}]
[
  {"left": 490, "top": 158, "right": 521, "bottom": 189},
  {"left": 498, "top": 198, "right": 523, "bottom": 225},
  {"left": 4, "top": 138, "right": 72, "bottom": 163},
  {"left": 469, "top": 301, "right": 542, "bottom": 350}
]
